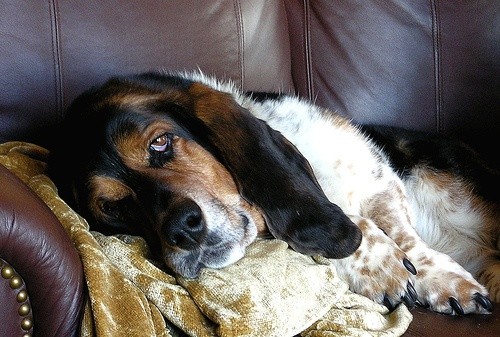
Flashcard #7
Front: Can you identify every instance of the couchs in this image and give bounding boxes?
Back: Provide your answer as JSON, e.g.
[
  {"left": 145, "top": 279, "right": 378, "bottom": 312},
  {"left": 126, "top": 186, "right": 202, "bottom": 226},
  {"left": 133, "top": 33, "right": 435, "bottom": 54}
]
[{"left": 0, "top": 0, "right": 500, "bottom": 336}]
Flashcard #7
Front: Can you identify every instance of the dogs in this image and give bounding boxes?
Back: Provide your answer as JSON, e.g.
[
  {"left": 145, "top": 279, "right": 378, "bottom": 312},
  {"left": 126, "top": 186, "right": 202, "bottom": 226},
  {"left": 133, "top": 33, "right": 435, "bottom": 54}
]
[{"left": 44, "top": 66, "right": 500, "bottom": 317}]
